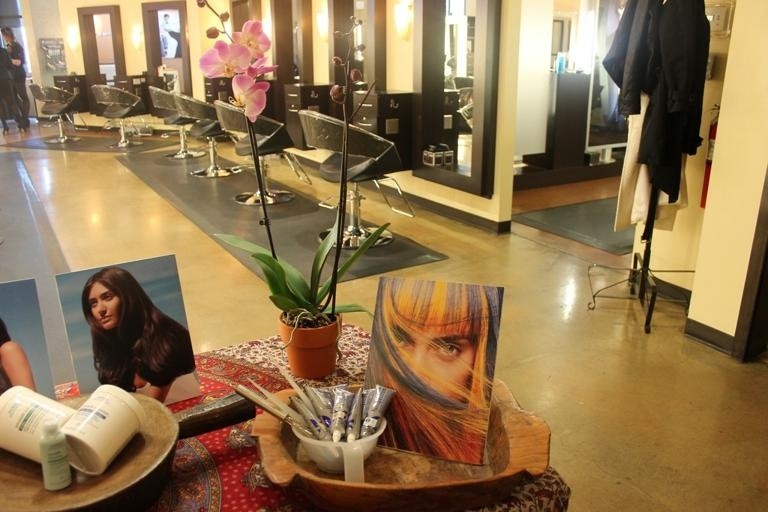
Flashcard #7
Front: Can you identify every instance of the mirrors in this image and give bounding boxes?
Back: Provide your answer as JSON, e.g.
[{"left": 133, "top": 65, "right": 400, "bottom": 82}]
[
  {"left": 585, "top": 0, "right": 631, "bottom": 155},
  {"left": 144, "top": 1, "right": 192, "bottom": 94},
  {"left": 414, "top": 0, "right": 494, "bottom": 196},
  {"left": 78, "top": 5, "right": 126, "bottom": 109},
  {"left": 269, "top": 0, "right": 314, "bottom": 101},
  {"left": 326, "top": 0, "right": 387, "bottom": 116}
]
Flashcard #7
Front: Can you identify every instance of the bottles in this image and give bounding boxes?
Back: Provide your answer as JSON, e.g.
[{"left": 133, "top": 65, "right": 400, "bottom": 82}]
[
  {"left": 38, "top": 421, "right": 71, "bottom": 493},
  {"left": 556, "top": 52, "right": 566, "bottom": 74}
]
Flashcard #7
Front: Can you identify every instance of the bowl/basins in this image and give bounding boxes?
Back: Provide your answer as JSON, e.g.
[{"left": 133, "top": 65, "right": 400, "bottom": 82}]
[{"left": 291, "top": 416, "right": 388, "bottom": 473}]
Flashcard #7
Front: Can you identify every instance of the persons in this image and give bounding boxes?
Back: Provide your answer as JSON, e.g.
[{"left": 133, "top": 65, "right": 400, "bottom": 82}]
[
  {"left": 0, "top": 49, "right": 28, "bottom": 135},
  {"left": 363, "top": 276, "right": 504, "bottom": 465},
  {"left": 0, "top": 318, "right": 35, "bottom": 396},
  {"left": 81, "top": 266, "right": 201, "bottom": 407},
  {"left": 1, "top": 28, "right": 30, "bottom": 127}
]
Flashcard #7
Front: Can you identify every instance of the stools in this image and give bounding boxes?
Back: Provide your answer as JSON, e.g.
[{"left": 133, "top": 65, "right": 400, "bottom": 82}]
[
  {"left": 214, "top": 97, "right": 296, "bottom": 204},
  {"left": 28, "top": 82, "right": 87, "bottom": 143},
  {"left": 299, "top": 108, "right": 396, "bottom": 249},
  {"left": 175, "top": 94, "right": 240, "bottom": 178},
  {"left": 150, "top": 85, "right": 208, "bottom": 159},
  {"left": 92, "top": 84, "right": 144, "bottom": 148}
]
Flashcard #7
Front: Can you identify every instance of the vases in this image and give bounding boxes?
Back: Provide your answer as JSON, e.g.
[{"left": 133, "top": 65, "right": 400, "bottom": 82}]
[{"left": 279, "top": 314, "right": 344, "bottom": 380}]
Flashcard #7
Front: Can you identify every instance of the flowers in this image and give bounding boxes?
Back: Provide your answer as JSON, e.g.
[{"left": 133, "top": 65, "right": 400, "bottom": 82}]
[{"left": 195, "top": 0, "right": 391, "bottom": 324}]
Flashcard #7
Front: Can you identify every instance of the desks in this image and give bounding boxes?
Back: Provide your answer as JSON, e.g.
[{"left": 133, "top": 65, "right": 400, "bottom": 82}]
[{"left": 45, "top": 321, "right": 573, "bottom": 512}]
